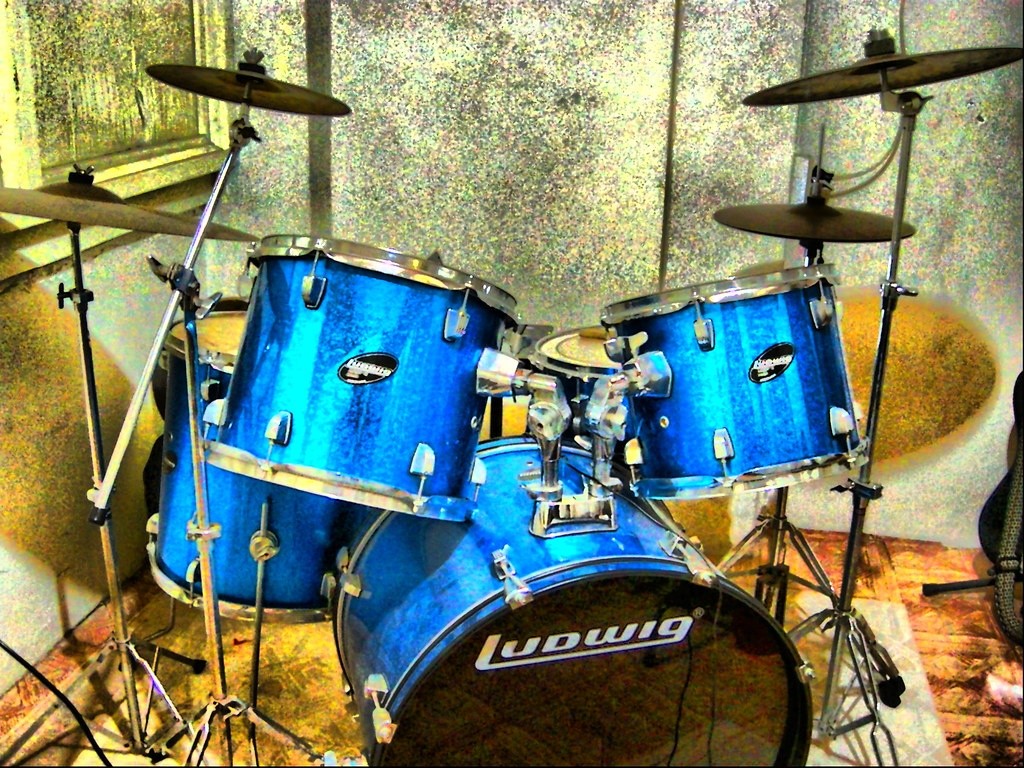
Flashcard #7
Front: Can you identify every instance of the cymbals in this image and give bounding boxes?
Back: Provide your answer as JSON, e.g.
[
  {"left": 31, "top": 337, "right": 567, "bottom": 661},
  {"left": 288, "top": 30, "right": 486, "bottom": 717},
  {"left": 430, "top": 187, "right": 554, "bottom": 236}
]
[
  {"left": 144, "top": 63, "right": 353, "bottom": 118},
  {"left": 712, "top": 203, "right": 918, "bottom": 245},
  {"left": 742, "top": 46, "right": 1024, "bottom": 108},
  {"left": 0, "top": 180, "right": 262, "bottom": 245}
]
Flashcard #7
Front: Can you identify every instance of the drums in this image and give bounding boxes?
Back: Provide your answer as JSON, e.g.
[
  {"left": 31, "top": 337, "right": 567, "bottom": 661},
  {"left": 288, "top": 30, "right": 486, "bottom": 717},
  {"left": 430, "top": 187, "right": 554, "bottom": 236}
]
[
  {"left": 204, "top": 233, "right": 517, "bottom": 524},
  {"left": 532, "top": 324, "right": 624, "bottom": 443},
  {"left": 598, "top": 262, "right": 870, "bottom": 501},
  {"left": 320, "top": 435, "right": 815, "bottom": 768},
  {"left": 147, "top": 310, "right": 369, "bottom": 626}
]
